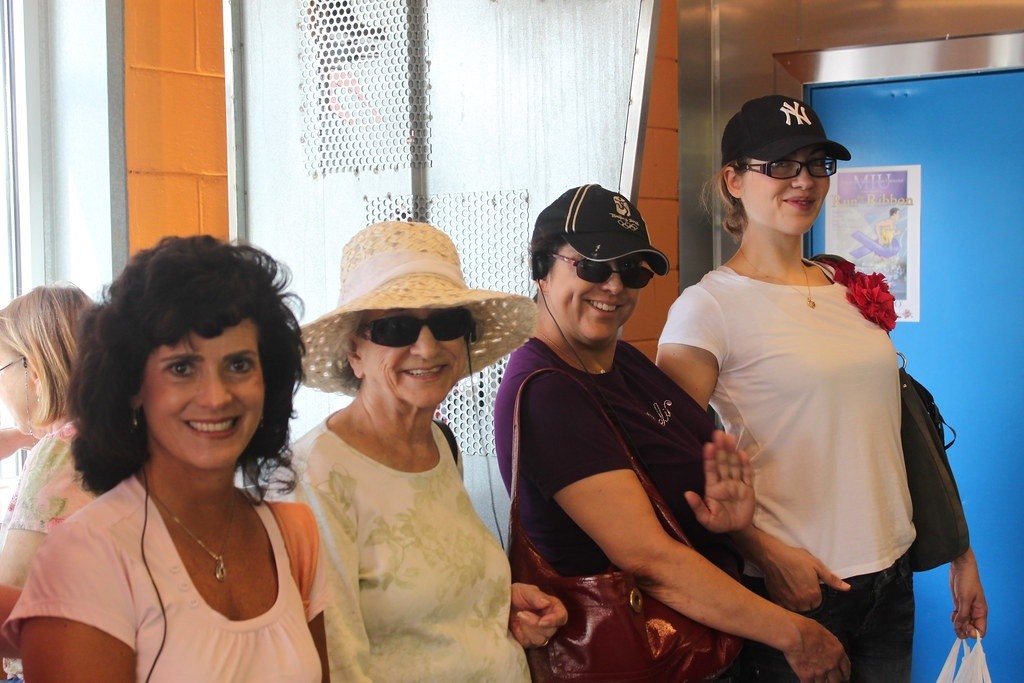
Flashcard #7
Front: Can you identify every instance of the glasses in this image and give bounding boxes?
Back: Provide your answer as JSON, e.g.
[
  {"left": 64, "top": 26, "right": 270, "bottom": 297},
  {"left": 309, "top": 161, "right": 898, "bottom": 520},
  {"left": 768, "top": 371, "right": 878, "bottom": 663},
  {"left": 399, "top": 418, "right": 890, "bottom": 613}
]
[
  {"left": 736, "top": 157, "right": 838, "bottom": 180},
  {"left": 356, "top": 307, "right": 476, "bottom": 348},
  {"left": 545, "top": 251, "right": 655, "bottom": 289}
]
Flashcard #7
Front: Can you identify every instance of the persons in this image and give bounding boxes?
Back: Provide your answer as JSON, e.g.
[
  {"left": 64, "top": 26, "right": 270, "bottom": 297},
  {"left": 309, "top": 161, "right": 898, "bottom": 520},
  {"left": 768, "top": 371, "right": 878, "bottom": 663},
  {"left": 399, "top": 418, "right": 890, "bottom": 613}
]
[
  {"left": 493, "top": 184, "right": 852, "bottom": 683},
  {"left": 0, "top": 282, "right": 97, "bottom": 683},
  {"left": 0, "top": 233, "right": 333, "bottom": 683},
  {"left": 255, "top": 219, "right": 568, "bottom": 683},
  {"left": 655, "top": 95, "right": 989, "bottom": 683}
]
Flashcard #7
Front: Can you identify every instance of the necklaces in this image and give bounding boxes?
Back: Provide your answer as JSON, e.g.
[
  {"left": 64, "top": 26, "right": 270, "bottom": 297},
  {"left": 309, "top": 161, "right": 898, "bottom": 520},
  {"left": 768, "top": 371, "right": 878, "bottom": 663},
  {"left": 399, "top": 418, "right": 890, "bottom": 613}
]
[
  {"left": 147, "top": 481, "right": 236, "bottom": 580},
  {"left": 532, "top": 330, "right": 615, "bottom": 375},
  {"left": 739, "top": 247, "right": 817, "bottom": 308}
]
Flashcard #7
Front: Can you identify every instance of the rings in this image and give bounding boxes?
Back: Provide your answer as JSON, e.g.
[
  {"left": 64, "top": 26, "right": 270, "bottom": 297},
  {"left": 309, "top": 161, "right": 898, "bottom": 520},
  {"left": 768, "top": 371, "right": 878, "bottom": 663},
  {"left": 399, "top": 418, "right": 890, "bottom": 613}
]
[{"left": 543, "top": 640, "right": 548, "bottom": 647}]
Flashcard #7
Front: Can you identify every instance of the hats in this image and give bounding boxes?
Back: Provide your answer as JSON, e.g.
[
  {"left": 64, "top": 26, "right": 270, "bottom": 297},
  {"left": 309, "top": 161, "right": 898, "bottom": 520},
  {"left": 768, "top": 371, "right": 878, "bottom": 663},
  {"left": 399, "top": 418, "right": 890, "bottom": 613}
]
[
  {"left": 530, "top": 183, "right": 671, "bottom": 276},
  {"left": 721, "top": 95, "right": 851, "bottom": 166},
  {"left": 279, "top": 220, "right": 538, "bottom": 398}
]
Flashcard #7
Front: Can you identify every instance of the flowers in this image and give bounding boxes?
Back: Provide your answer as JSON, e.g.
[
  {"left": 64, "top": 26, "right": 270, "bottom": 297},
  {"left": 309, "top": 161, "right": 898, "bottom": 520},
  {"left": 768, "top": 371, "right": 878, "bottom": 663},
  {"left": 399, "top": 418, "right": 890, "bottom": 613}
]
[{"left": 822, "top": 257, "right": 899, "bottom": 333}]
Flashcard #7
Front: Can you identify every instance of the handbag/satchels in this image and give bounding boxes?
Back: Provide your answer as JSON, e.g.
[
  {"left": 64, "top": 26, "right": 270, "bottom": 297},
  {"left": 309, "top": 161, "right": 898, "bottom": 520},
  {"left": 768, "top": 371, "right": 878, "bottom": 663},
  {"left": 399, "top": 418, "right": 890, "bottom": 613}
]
[
  {"left": 935, "top": 629, "right": 990, "bottom": 683},
  {"left": 890, "top": 350, "right": 970, "bottom": 574},
  {"left": 500, "top": 365, "right": 744, "bottom": 683}
]
